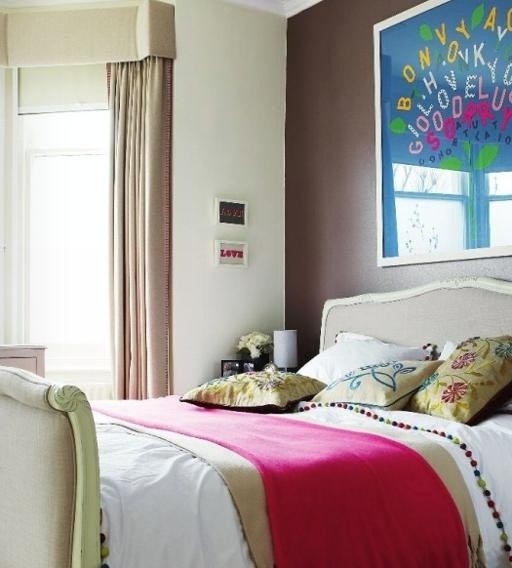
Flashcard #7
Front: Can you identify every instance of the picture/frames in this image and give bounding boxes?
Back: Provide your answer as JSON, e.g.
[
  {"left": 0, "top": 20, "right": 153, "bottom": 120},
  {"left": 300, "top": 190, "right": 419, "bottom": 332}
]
[
  {"left": 221, "top": 360, "right": 242, "bottom": 376},
  {"left": 215, "top": 240, "right": 248, "bottom": 268},
  {"left": 372, "top": 0, "right": 512, "bottom": 267},
  {"left": 215, "top": 198, "right": 247, "bottom": 228}
]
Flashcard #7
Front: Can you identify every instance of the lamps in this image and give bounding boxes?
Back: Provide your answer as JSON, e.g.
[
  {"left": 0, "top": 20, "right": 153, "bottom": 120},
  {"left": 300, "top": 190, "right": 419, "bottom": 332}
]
[{"left": 273, "top": 330, "right": 299, "bottom": 372}]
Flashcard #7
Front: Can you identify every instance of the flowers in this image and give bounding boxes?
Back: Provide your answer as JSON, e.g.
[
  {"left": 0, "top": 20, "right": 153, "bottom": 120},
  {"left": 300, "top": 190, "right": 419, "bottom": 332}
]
[{"left": 237, "top": 332, "right": 274, "bottom": 360}]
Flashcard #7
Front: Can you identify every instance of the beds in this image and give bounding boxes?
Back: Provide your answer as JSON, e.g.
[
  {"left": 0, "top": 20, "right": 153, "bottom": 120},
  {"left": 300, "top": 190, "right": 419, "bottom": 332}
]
[{"left": 0, "top": 275, "right": 512, "bottom": 568}]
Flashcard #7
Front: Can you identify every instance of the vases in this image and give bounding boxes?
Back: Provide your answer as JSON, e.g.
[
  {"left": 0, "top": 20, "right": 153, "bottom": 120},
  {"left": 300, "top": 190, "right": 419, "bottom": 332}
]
[{"left": 245, "top": 354, "right": 269, "bottom": 371}]
